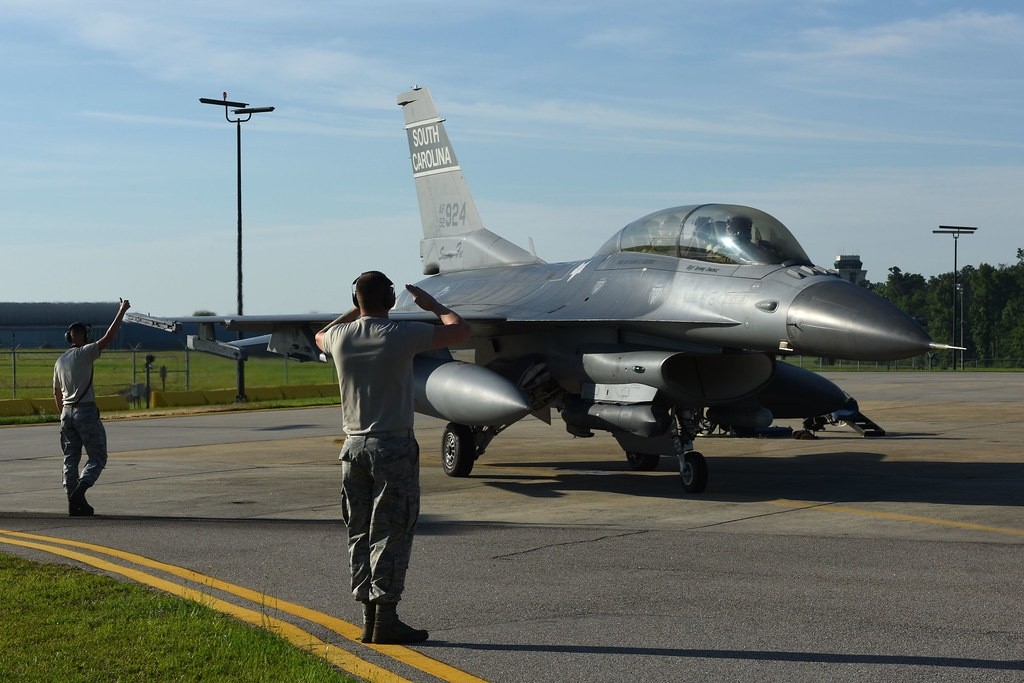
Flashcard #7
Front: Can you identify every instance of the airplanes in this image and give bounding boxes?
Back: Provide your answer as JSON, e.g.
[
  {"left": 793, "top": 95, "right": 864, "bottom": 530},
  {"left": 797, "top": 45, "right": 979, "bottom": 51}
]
[{"left": 119, "top": 83, "right": 968, "bottom": 496}]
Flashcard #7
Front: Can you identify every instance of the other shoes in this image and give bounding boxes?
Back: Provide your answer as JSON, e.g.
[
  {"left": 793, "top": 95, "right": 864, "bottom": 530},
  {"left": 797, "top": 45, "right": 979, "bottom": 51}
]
[{"left": 69, "top": 494, "right": 94, "bottom": 515}]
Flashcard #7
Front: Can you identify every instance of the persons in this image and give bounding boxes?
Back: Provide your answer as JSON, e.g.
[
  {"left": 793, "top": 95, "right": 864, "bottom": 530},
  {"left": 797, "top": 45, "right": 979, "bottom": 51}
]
[
  {"left": 52, "top": 297, "right": 131, "bottom": 515},
  {"left": 314, "top": 270, "right": 473, "bottom": 644},
  {"left": 643, "top": 214, "right": 777, "bottom": 265}
]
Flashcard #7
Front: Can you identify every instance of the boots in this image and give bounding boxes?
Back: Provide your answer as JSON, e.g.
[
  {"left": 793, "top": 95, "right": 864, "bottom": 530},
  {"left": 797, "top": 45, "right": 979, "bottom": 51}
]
[
  {"left": 372, "top": 603, "right": 429, "bottom": 644},
  {"left": 361, "top": 603, "right": 374, "bottom": 642}
]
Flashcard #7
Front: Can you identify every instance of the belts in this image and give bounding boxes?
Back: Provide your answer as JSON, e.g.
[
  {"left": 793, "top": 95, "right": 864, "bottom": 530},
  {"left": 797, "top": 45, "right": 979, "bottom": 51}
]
[
  {"left": 64, "top": 402, "right": 95, "bottom": 407},
  {"left": 366, "top": 428, "right": 415, "bottom": 438}
]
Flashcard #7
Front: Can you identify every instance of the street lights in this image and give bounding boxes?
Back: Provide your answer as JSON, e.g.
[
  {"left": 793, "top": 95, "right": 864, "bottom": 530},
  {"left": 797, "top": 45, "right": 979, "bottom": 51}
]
[
  {"left": 953, "top": 282, "right": 965, "bottom": 372},
  {"left": 195, "top": 88, "right": 278, "bottom": 401},
  {"left": 931, "top": 225, "right": 979, "bottom": 369}
]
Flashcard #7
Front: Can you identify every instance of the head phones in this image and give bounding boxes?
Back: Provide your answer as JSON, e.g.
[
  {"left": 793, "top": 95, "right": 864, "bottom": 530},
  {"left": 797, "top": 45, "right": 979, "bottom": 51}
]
[
  {"left": 351, "top": 271, "right": 396, "bottom": 310},
  {"left": 65, "top": 323, "right": 88, "bottom": 343}
]
